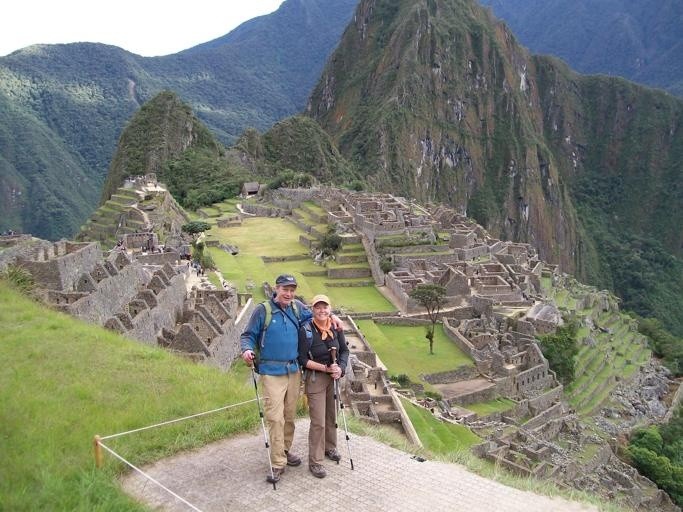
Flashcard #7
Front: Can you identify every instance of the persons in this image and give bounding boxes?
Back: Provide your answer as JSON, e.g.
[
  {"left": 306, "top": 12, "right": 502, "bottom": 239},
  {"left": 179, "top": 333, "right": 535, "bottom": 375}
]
[
  {"left": 239, "top": 273, "right": 344, "bottom": 483},
  {"left": 297, "top": 293, "right": 349, "bottom": 478},
  {"left": 116, "top": 236, "right": 203, "bottom": 280}
]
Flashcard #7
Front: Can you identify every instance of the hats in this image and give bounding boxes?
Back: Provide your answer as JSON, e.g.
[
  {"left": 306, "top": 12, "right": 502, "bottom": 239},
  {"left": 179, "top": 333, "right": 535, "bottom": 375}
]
[
  {"left": 276, "top": 274, "right": 296, "bottom": 288},
  {"left": 313, "top": 294, "right": 329, "bottom": 306}
]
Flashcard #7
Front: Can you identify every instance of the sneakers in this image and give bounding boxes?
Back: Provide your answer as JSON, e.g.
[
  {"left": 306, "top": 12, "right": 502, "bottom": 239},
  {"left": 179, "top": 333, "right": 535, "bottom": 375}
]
[
  {"left": 325, "top": 449, "right": 341, "bottom": 461},
  {"left": 308, "top": 463, "right": 327, "bottom": 478},
  {"left": 285, "top": 452, "right": 301, "bottom": 464},
  {"left": 267, "top": 469, "right": 285, "bottom": 481}
]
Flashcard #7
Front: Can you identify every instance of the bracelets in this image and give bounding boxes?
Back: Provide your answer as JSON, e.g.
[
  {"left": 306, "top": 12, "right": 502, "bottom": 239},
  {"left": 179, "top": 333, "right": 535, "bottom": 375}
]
[
  {"left": 328, "top": 313, "right": 334, "bottom": 318},
  {"left": 325, "top": 364, "right": 328, "bottom": 372}
]
